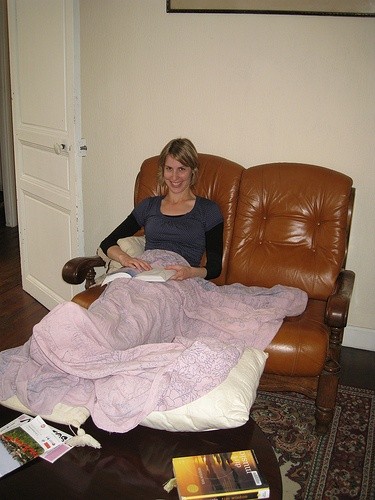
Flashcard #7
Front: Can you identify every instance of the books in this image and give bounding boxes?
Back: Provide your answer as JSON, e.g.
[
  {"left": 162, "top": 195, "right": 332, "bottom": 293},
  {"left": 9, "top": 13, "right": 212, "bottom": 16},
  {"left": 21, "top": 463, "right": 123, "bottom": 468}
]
[
  {"left": 0, "top": 415, "right": 62, "bottom": 480},
  {"left": 172, "top": 449, "right": 270, "bottom": 500},
  {"left": 101, "top": 266, "right": 176, "bottom": 287}
]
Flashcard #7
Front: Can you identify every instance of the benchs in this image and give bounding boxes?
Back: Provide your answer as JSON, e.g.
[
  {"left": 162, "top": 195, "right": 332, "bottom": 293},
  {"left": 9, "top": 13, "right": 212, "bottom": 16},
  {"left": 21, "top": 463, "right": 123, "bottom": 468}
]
[{"left": 61, "top": 153, "right": 355, "bottom": 436}]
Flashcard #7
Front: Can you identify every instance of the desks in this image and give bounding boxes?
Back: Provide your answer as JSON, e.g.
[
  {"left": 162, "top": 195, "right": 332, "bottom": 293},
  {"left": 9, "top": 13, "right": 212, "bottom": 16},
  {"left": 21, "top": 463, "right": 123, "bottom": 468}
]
[{"left": 0, "top": 404, "right": 283, "bottom": 500}]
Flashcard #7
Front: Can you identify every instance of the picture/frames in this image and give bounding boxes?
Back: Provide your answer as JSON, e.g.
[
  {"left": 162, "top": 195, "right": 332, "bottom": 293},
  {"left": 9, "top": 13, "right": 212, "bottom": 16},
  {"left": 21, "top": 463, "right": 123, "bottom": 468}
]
[{"left": 166, "top": 0, "right": 375, "bottom": 18}]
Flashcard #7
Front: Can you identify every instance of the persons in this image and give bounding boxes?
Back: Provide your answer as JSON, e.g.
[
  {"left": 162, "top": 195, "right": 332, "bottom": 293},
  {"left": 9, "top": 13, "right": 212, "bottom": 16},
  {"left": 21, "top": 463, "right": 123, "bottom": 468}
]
[{"left": 99, "top": 138, "right": 225, "bottom": 281}]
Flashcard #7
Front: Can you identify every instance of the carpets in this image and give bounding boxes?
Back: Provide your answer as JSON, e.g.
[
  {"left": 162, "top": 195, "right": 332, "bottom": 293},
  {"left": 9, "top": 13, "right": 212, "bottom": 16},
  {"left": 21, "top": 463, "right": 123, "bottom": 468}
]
[{"left": 250, "top": 383, "right": 375, "bottom": 500}]
